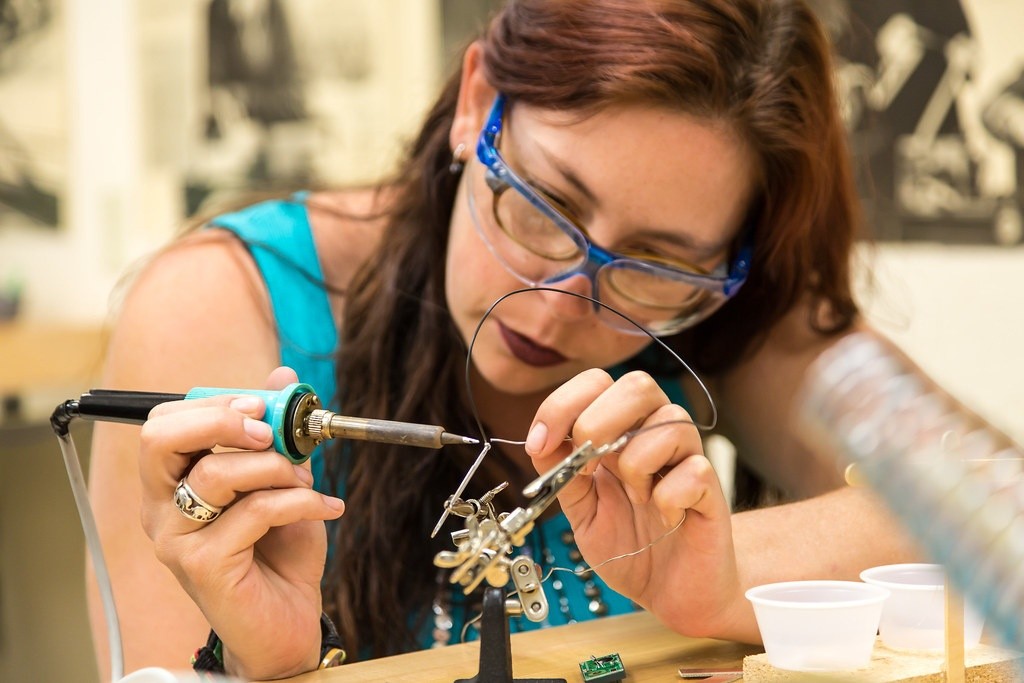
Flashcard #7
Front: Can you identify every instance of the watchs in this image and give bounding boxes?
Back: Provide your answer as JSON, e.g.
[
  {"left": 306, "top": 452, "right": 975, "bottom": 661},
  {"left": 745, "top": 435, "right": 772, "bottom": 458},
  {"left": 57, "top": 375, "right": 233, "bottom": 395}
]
[{"left": 192, "top": 610, "right": 346, "bottom": 681}]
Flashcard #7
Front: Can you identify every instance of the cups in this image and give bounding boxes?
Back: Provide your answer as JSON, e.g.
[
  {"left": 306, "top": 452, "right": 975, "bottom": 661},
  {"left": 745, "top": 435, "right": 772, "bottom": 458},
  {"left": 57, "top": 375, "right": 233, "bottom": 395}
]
[
  {"left": 745, "top": 580, "right": 888, "bottom": 671},
  {"left": 860, "top": 564, "right": 985, "bottom": 654}
]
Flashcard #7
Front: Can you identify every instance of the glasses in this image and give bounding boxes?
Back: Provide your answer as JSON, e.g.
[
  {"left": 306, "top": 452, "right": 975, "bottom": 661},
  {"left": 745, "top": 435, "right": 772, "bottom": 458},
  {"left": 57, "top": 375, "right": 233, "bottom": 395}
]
[{"left": 465, "top": 92, "right": 754, "bottom": 337}]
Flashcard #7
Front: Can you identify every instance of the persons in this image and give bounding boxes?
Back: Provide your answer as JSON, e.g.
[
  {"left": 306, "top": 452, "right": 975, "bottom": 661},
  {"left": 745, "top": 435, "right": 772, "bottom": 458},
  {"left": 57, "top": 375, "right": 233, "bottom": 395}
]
[{"left": 82, "top": 1, "right": 925, "bottom": 683}]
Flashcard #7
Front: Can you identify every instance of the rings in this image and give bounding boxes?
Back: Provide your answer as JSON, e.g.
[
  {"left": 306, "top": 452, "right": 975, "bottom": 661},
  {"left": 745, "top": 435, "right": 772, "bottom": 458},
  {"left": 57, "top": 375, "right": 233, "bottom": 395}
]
[{"left": 174, "top": 475, "right": 223, "bottom": 523}]
